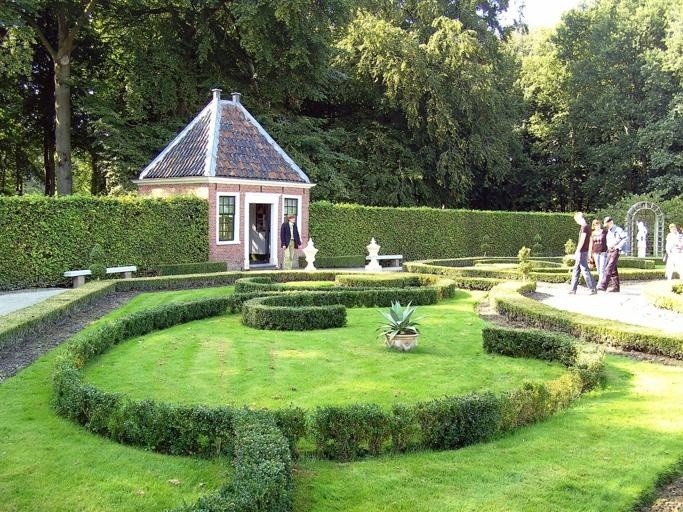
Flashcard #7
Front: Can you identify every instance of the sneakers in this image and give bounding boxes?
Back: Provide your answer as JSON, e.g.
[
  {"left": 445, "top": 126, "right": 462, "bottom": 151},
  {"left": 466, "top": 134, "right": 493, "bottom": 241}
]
[{"left": 565, "top": 282, "right": 624, "bottom": 297}]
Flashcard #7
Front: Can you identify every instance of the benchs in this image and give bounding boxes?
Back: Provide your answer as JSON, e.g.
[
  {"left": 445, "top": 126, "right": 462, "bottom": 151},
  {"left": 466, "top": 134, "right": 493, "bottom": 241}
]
[
  {"left": 65, "top": 266, "right": 136, "bottom": 289},
  {"left": 366, "top": 255, "right": 402, "bottom": 267}
]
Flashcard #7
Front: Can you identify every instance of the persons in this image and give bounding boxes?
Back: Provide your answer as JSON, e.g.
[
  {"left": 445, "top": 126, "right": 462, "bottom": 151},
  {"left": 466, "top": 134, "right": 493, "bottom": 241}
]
[
  {"left": 281, "top": 214, "right": 302, "bottom": 269},
  {"left": 597, "top": 217, "right": 628, "bottom": 292},
  {"left": 588, "top": 219, "right": 608, "bottom": 289},
  {"left": 663, "top": 223, "right": 683, "bottom": 280},
  {"left": 568, "top": 211, "right": 598, "bottom": 295}
]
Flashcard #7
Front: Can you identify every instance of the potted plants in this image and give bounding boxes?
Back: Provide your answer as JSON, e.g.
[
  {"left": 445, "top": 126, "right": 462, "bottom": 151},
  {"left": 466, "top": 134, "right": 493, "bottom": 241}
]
[{"left": 373, "top": 300, "right": 424, "bottom": 352}]
